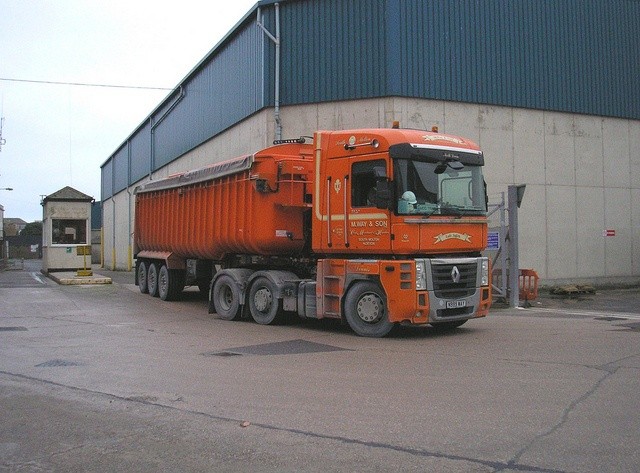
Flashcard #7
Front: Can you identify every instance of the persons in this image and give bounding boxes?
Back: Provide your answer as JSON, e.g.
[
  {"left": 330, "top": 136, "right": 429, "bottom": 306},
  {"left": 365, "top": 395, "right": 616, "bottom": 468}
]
[{"left": 367, "top": 179, "right": 389, "bottom": 205}]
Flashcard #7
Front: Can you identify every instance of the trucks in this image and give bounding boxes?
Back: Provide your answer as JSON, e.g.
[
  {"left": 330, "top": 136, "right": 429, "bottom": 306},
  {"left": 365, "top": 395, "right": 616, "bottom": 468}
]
[{"left": 133, "top": 121, "right": 491, "bottom": 338}]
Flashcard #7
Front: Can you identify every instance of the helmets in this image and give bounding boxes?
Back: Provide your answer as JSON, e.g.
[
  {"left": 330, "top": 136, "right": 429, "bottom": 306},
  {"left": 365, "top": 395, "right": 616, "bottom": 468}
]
[{"left": 402, "top": 191, "right": 416, "bottom": 202}]
